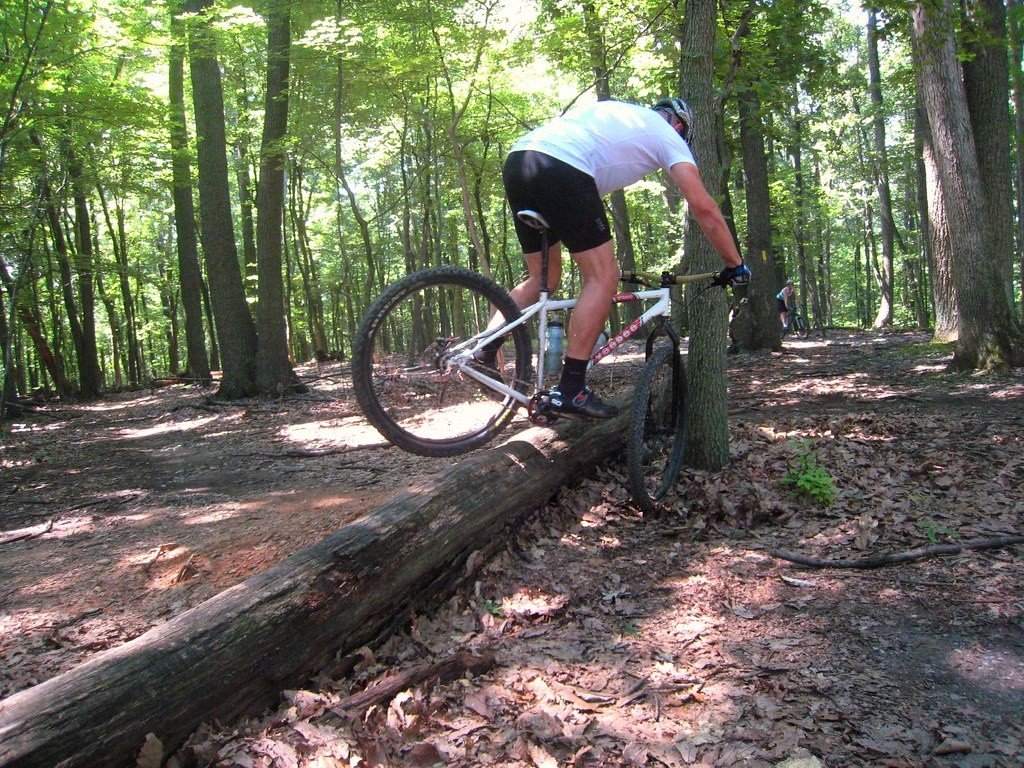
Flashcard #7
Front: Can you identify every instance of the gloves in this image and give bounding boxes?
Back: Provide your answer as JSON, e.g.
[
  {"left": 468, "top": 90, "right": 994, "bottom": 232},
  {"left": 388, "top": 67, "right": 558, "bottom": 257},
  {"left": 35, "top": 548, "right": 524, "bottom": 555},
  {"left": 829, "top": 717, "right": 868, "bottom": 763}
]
[{"left": 728, "top": 261, "right": 751, "bottom": 285}]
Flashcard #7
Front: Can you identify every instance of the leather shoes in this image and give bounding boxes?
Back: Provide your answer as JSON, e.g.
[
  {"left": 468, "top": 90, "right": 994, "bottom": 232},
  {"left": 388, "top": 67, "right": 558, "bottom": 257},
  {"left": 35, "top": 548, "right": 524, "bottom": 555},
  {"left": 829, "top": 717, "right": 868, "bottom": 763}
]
[
  {"left": 460, "top": 351, "right": 506, "bottom": 402},
  {"left": 547, "top": 379, "right": 619, "bottom": 418}
]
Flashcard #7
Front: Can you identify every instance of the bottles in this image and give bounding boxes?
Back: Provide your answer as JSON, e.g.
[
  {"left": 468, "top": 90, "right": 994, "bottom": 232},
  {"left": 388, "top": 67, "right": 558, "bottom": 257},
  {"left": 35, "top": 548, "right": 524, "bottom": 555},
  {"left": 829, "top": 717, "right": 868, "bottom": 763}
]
[
  {"left": 546, "top": 314, "right": 563, "bottom": 375},
  {"left": 588, "top": 327, "right": 612, "bottom": 357}
]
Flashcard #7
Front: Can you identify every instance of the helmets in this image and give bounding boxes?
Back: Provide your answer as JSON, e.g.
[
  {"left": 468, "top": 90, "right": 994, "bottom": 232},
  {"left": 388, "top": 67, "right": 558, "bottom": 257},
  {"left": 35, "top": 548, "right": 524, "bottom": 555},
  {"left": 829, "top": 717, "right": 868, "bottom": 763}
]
[{"left": 652, "top": 97, "right": 695, "bottom": 149}]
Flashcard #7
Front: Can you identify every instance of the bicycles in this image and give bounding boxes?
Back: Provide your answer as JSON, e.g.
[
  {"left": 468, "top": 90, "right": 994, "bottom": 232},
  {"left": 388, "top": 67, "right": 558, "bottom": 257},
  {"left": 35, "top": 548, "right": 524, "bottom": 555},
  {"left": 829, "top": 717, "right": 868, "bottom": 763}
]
[
  {"left": 777, "top": 304, "right": 811, "bottom": 340},
  {"left": 353, "top": 208, "right": 747, "bottom": 516}
]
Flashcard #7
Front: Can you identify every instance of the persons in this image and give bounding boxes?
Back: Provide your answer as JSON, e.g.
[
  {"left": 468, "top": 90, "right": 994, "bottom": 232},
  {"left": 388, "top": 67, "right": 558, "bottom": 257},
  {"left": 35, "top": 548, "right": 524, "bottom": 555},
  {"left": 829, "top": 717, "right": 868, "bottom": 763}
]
[
  {"left": 775, "top": 279, "right": 810, "bottom": 330},
  {"left": 455, "top": 94, "right": 753, "bottom": 424}
]
